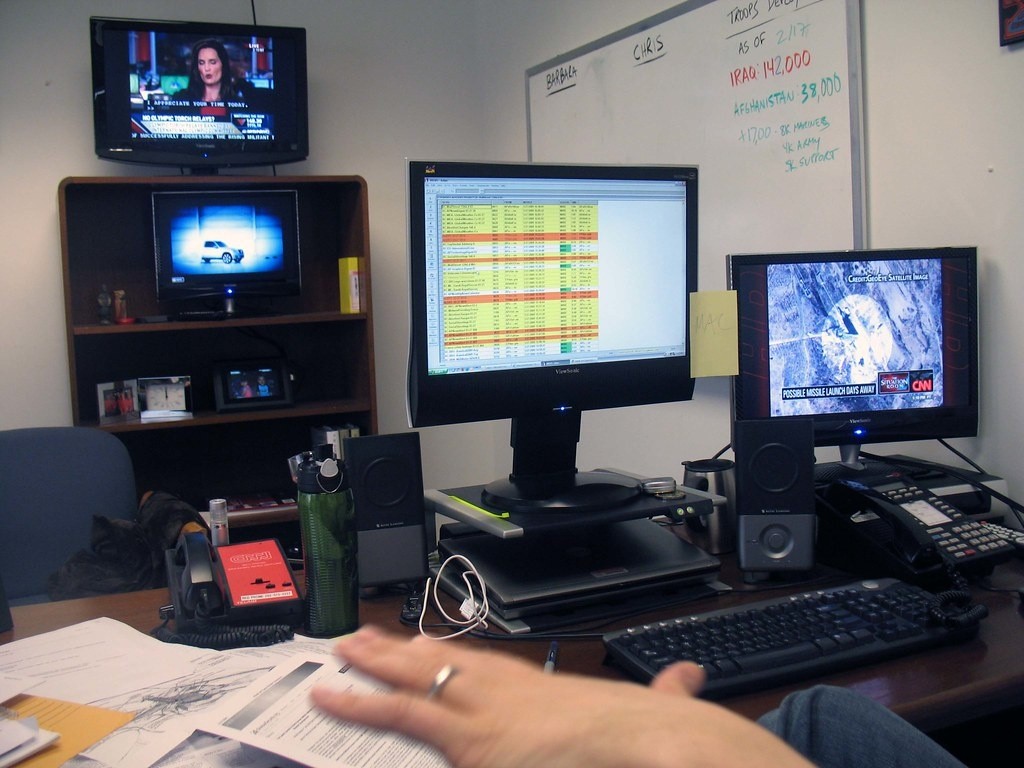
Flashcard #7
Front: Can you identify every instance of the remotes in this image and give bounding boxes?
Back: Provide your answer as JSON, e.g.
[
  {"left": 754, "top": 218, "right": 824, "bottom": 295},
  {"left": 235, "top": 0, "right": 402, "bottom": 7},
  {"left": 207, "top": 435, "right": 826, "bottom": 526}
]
[
  {"left": 981, "top": 520, "right": 1024, "bottom": 552},
  {"left": 401, "top": 594, "right": 422, "bottom": 622}
]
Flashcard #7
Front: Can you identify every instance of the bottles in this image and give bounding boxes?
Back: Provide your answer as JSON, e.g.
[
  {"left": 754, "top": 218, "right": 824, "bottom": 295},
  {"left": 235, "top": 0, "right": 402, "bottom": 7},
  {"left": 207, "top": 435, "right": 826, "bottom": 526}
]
[{"left": 297, "top": 442, "right": 360, "bottom": 639}]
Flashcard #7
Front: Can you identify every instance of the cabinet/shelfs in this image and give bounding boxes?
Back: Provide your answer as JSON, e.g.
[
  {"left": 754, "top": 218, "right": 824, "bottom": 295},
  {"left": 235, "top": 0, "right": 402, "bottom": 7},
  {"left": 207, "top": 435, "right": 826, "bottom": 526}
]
[{"left": 56, "top": 172, "right": 378, "bottom": 534}]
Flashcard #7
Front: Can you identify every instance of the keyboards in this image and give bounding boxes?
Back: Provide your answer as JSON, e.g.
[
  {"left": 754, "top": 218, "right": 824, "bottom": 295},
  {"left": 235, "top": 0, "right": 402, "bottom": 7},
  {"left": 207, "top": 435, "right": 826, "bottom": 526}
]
[{"left": 602, "top": 577, "right": 977, "bottom": 700}]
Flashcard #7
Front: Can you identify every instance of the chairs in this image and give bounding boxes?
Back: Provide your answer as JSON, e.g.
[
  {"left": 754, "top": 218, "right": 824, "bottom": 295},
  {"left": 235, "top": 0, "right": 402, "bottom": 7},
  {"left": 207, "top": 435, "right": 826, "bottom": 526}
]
[{"left": 1, "top": 427, "right": 140, "bottom": 601}]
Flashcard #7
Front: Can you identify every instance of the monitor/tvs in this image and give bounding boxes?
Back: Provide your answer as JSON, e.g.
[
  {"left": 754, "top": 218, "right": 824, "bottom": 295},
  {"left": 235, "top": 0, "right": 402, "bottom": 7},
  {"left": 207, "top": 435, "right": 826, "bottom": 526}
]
[
  {"left": 726, "top": 247, "right": 979, "bottom": 490},
  {"left": 149, "top": 189, "right": 309, "bottom": 318},
  {"left": 88, "top": 16, "right": 309, "bottom": 178},
  {"left": 408, "top": 161, "right": 697, "bottom": 514}
]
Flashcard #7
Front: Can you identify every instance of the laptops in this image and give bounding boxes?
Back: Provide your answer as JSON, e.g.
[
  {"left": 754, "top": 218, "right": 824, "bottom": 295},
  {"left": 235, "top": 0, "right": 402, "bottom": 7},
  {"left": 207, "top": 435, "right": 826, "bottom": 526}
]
[{"left": 437, "top": 518, "right": 722, "bottom": 621}]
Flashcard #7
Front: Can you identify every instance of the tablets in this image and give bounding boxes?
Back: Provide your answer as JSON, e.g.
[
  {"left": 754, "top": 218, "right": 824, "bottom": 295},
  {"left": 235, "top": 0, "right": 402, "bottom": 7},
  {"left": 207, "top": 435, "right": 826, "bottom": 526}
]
[{"left": 214, "top": 358, "right": 296, "bottom": 412}]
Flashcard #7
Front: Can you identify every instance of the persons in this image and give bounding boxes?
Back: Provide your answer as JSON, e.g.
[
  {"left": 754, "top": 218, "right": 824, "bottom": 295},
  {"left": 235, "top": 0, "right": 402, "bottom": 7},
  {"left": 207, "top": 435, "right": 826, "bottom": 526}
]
[
  {"left": 170, "top": 38, "right": 243, "bottom": 119},
  {"left": 97, "top": 283, "right": 111, "bottom": 322},
  {"left": 105, "top": 391, "right": 133, "bottom": 412},
  {"left": 311, "top": 622, "right": 970, "bottom": 768},
  {"left": 240, "top": 375, "right": 272, "bottom": 399}
]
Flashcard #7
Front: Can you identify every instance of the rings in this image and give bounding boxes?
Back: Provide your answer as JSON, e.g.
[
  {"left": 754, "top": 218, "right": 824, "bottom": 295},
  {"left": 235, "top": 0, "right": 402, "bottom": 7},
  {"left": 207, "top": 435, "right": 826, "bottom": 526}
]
[{"left": 430, "top": 664, "right": 452, "bottom": 697}]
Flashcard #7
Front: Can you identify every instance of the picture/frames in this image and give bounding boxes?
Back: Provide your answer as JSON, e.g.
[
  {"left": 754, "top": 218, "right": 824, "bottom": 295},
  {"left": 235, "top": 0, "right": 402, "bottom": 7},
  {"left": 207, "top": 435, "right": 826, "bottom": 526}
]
[{"left": 97, "top": 380, "right": 139, "bottom": 425}]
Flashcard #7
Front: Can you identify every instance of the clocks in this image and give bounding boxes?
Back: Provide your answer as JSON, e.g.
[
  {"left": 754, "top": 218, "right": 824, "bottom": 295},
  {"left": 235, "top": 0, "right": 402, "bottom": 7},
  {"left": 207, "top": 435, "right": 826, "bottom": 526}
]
[{"left": 137, "top": 375, "right": 194, "bottom": 418}]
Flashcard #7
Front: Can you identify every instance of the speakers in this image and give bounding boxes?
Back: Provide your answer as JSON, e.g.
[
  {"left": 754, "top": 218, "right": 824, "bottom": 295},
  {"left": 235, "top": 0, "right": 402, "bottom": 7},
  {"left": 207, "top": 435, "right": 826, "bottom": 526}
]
[
  {"left": 343, "top": 432, "right": 430, "bottom": 586},
  {"left": 734, "top": 418, "right": 818, "bottom": 584}
]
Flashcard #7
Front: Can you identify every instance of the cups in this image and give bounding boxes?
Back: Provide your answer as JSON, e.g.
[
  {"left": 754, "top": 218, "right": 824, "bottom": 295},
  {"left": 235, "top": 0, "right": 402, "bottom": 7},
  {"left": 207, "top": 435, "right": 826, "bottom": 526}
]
[{"left": 681, "top": 458, "right": 737, "bottom": 556}]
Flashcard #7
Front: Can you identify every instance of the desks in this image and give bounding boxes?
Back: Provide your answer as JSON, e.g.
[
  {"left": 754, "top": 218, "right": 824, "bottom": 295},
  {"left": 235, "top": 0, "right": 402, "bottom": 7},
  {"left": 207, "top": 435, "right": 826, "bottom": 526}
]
[{"left": 1, "top": 555, "right": 1024, "bottom": 766}]
